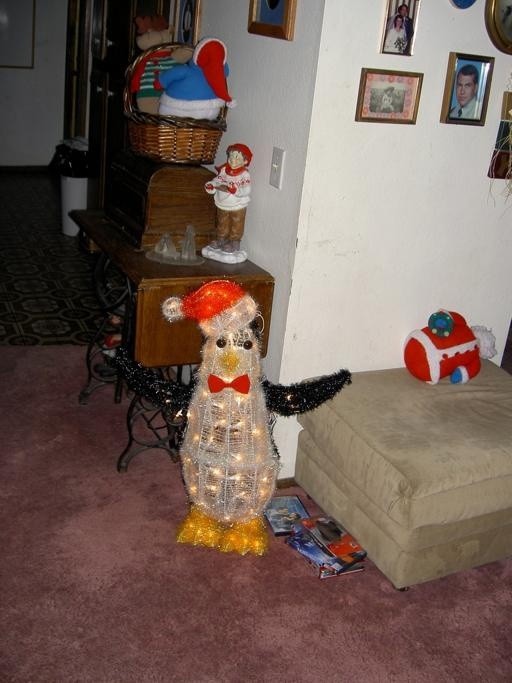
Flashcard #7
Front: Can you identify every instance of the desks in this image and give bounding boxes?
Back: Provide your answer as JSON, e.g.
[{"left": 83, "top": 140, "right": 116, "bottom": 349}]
[{"left": 68, "top": 209, "right": 275, "bottom": 472}]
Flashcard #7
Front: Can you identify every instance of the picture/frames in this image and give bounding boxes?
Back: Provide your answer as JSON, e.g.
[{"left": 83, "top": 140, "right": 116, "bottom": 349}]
[
  {"left": 439, "top": 52, "right": 495, "bottom": 126},
  {"left": 380, "top": 0, "right": 421, "bottom": 56},
  {"left": 248, "top": 0, "right": 296, "bottom": 42},
  {"left": 172, "top": 0, "right": 201, "bottom": 49},
  {"left": 355, "top": 68, "right": 424, "bottom": 124}
]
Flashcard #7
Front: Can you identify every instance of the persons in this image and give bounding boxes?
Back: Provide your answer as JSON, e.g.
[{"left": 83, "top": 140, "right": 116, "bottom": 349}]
[
  {"left": 387, "top": 3, "right": 415, "bottom": 55},
  {"left": 380, "top": 85, "right": 397, "bottom": 110},
  {"left": 385, "top": 13, "right": 408, "bottom": 53},
  {"left": 198, "top": 142, "right": 255, "bottom": 265},
  {"left": 445, "top": 60, "right": 483, "bottom": 123}
]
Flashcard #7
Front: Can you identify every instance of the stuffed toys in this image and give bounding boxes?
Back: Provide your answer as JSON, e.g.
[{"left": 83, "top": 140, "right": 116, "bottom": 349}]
[
  {"left": 150, "top": 36, "right": 238, "bottom": 122},
  {"left": 127, "top": 11, "right": 197, "bottom": 116},
  {"left": 101, "top": 275, "right": 355, "bottom": 561},
  {"left": 403, "top": 306, "right": 500, "bottom": 386}
]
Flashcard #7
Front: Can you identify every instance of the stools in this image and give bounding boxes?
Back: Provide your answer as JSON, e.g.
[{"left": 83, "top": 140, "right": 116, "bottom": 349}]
[{"left": 293, "top": 361, "right": 512, "bottom": 593}]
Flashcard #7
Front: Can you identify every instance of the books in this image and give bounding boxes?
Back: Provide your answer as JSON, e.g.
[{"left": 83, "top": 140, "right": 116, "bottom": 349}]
[
  {"left": 284, "top": 526, "right": 368, "bottom": 575},
  {"left": 264, "top": 492, "right": 310, "bottom": 536},
  {"left": 298, "top": 513, "right": 363, "bottom": 558},
  {"left": 293, "top": 550, "right": 367, "bottom": 580}
]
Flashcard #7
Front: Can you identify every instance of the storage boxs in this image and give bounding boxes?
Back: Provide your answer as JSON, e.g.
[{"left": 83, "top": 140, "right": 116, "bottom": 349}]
[{"left": 103, "top": 153, "right": 218, "bottom": 253}]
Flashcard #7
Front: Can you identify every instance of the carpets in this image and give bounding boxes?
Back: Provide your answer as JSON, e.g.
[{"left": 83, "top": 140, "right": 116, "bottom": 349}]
[{"left": 0, "top": 177, "right": 137, "bottom": 347}]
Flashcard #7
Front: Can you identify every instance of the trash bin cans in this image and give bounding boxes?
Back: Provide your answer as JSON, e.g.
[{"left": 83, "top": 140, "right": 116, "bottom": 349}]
[{"left": 58, "top": 146, "right": 88, "bottom": 237}]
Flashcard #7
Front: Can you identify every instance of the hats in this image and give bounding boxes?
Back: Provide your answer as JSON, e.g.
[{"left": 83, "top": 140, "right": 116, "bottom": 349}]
[{"left": 193, "top": 36, "right": 241, "bottom": 112}]
